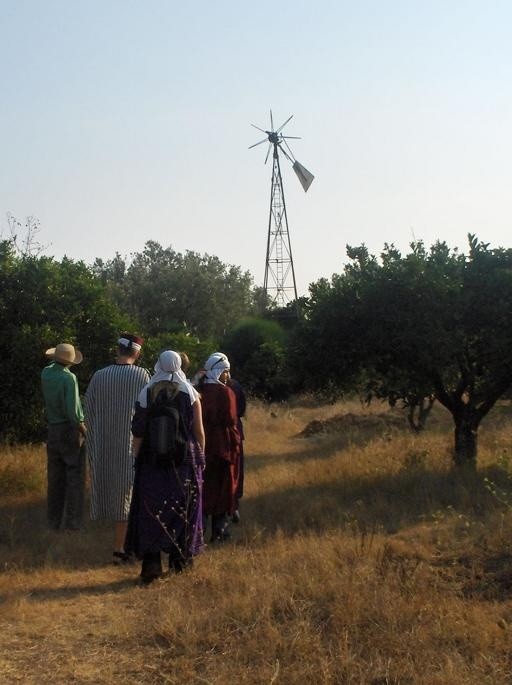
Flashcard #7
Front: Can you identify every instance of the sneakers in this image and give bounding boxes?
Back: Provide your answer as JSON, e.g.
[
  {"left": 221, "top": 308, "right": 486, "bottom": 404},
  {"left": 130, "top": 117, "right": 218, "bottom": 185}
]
[{"left": 49, "top": 509, "right": 240, "bottom": 586}]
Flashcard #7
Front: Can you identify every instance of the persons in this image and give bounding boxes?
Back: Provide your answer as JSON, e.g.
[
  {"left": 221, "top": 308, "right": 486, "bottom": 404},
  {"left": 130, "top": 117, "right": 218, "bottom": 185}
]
[
  {"left": 40, "top": 343, "right": 89, "bottom": 532},
  {"left": 210, "top": 352, "right": 247, "bottom": 524},
  {"left": 83, "top": 332, "right": 151, "bottom": 565},
  {"left": 191, "top": 356, "right": 241, "bottom": 542},
  {"left": 122, "top": 350, "right": 206, "bottom": 586}
]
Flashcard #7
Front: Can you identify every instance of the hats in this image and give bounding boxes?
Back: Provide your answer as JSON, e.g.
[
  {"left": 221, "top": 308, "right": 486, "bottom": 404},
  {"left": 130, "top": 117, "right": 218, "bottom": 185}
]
[
  {"left": 45, "top": 343, "right": 83, "bottom": 366},
  {"left": 119, "top": 332, "right": 144, "bottom": 351}
]
